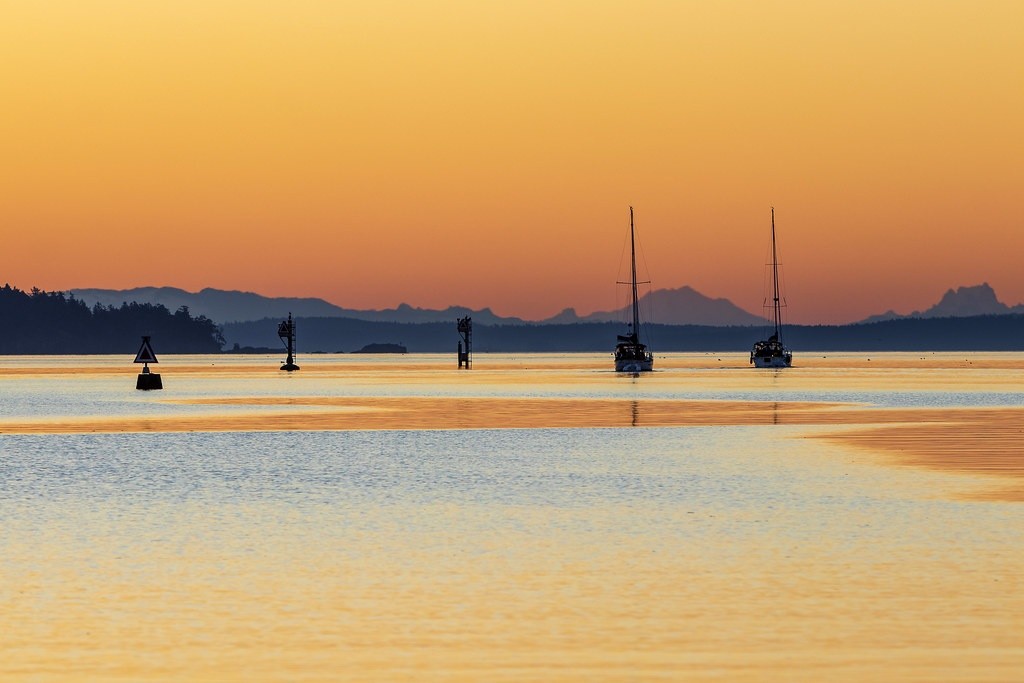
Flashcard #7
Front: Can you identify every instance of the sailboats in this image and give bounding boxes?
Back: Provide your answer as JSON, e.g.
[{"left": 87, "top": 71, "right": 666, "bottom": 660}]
[
  {"left": 748, "top": 207, "right": 793, "bottom": 367},
  {"left": 612, "top": 206, "right": 654, "bottom": 373}
]
